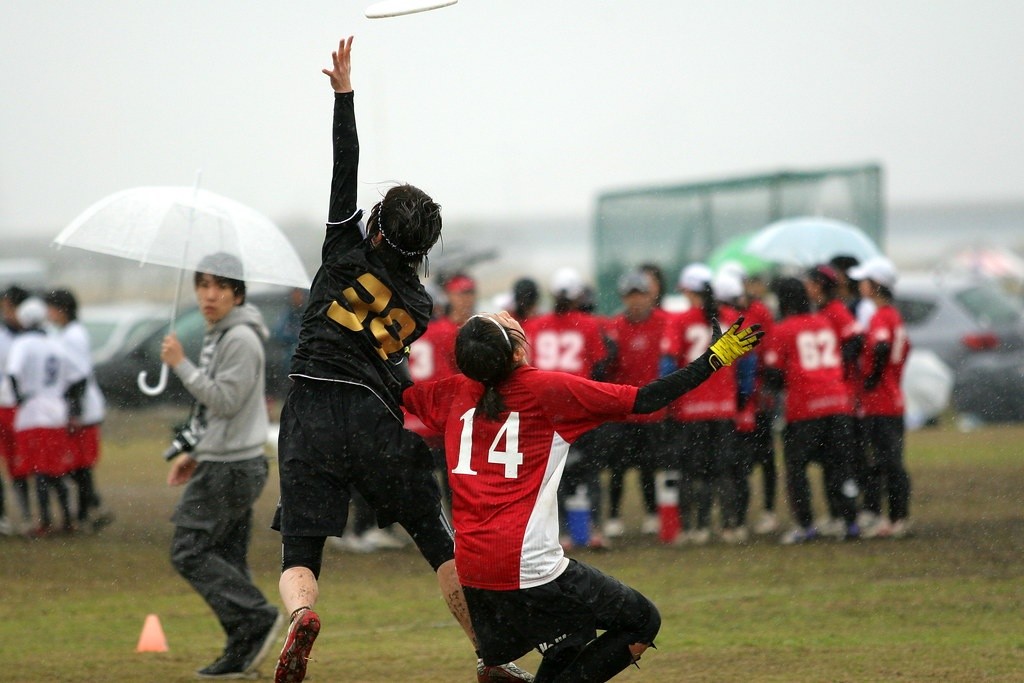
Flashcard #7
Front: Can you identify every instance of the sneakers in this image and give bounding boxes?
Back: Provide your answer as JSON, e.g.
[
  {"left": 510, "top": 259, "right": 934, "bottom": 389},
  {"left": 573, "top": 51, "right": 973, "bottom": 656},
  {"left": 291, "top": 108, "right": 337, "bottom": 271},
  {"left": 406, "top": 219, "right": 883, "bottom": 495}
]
[
  {"left": 476, "top": 657, "right": 535, "bottom": 683},
  {"left": 274, "top": 608, "right": 321, "bottom": 683}
]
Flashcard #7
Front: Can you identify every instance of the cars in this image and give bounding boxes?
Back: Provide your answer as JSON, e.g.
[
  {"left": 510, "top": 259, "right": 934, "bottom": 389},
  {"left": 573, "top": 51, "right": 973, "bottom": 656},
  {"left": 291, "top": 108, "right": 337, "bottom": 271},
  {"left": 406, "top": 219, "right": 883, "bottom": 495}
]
[
  {"left": 93, "top": 293, "right": 306, "bottom": 412},
  {"left": 890, "top": 273, "right": 1024, "bottom": 423},
  {"left": 75, "top": 301, "right": 192, "bottom": 375}
]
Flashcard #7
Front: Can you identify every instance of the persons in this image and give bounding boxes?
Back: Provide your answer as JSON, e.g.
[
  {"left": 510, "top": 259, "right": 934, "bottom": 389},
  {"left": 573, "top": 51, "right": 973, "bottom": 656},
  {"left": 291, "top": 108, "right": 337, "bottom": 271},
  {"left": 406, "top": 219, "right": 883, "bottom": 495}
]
[
  {"left": 160, "top": 252, "right": 282, "bottom": 679},
  {"left": 1, "top": 285, "right": 115, "bottom": 538},
  {"left": 272, "top": 34, "right": 535, "bottom": 683},
  {"left": 324, "top": 257, "right": 913, "bottom": 551},
  {"left": 402, "top": 311, "right": 766, "bottom": 683}
]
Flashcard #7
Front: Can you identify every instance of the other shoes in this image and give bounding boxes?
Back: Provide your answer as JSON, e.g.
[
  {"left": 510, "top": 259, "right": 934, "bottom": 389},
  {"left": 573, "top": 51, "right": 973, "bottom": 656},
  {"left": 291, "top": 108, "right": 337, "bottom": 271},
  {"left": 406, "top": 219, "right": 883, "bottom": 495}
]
[
  {"left": 196, "top": 656, "right": 246, "bottom": 679},
  {"left": 559, "top": 506, "right": 915, "bottom": 552},
  {"left": 244, "top": 606, "right": 280, "bottom": 672},
  {"left": 327, "top": 524, "right": 414, "bottom": 557}
]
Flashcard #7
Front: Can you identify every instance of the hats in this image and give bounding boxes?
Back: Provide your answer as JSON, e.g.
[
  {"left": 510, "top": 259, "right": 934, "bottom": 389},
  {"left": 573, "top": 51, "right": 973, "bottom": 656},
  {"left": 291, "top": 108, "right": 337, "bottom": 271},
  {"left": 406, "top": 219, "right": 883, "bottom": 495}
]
[
  {"left": 711, "top": 273, "right": 745, "bottom": 302},
  {"left": 800, "top": 263, "right": 841, "bottom": 294},
  {"left": 615, "top": 268, "right": 648, "bottom": 297},
  {"left": 677, "top": 262, "right": 714, "bottom": 292},
  {"left": 846, "top": 256, "right": 898, "bottom": 291},
  {"left": 443, "top": 274, "right": 474, "bottom": 293},
  {"left": 14, "top": 296, "right": 46, "bottom": 329},
  {"left": 548, "top": 267, "right": 578, "bottom": 301}
]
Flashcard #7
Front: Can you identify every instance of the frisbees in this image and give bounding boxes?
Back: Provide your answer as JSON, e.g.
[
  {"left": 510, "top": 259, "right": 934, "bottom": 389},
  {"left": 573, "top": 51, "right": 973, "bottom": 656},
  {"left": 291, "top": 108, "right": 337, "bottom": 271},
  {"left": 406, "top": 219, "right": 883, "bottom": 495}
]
[{"left": 365, "top": 0, "right": 458, "bottom": 19}]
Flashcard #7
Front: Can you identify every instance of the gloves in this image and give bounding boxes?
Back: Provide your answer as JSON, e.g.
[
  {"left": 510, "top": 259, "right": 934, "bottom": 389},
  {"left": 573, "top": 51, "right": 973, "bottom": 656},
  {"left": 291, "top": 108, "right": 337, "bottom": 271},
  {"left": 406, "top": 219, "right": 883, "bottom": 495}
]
[{"left": 708, "top": 315, "right": 766, "bottom": 367}]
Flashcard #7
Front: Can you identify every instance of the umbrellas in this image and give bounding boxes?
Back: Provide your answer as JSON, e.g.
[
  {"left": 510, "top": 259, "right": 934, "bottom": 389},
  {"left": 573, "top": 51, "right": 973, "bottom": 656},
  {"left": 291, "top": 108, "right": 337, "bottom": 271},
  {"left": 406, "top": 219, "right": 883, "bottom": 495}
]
[
  {"left": 704, "top": 212, "right": 889, "bottom": 279},
  {"left": 54, "top": 172, "right": 313, "bottom": 396},
  {"left": 899, "top": 339, "right": 1024, "bottom": 429}
]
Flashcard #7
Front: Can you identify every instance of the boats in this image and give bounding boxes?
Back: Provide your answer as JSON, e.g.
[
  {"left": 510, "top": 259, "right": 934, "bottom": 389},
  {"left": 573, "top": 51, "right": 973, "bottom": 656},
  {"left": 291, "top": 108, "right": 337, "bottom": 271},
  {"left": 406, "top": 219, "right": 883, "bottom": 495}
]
[{"left": 138, "top": 613, "right": 172, "bottom": 655}]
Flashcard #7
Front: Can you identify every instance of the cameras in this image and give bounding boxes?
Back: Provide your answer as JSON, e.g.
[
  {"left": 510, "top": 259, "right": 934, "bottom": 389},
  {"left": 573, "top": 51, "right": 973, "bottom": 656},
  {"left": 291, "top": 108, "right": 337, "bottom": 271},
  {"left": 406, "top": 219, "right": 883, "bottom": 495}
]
[{"left": 163, "top": 422, "right": 199, "bottom": 462}]
